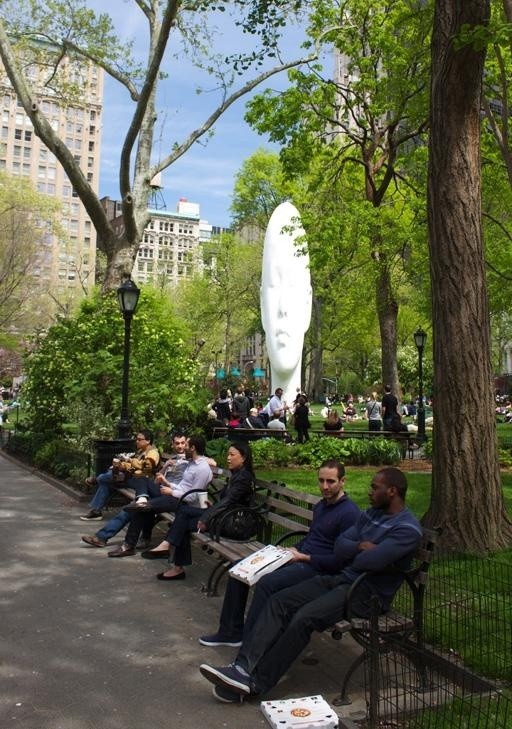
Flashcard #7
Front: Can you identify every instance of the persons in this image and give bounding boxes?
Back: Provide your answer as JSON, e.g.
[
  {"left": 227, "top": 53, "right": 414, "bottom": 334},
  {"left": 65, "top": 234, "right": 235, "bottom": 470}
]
[
  {"left": 108, "top": 434, "right": 214, "bottom": 558},
  {"left": 209, "top": 383, "right": 433, "bottom": 451},
  {"left": 197, "top": 460, "right": 359, "bottom": 648},
  {"left": 258, "top": 201, "right": 314, "bottom": 414},
  {"left": 79, "top": 429, "right": 161, "bottom": 521},
  {"left": 198, "top": 468, "right": 424, "bottom": 704},
  {"left": 139, "top": 439, "right": 255, "bottom": 581},
  {"left": 78, "top": 432, "right": 217, "bottom": 550}
]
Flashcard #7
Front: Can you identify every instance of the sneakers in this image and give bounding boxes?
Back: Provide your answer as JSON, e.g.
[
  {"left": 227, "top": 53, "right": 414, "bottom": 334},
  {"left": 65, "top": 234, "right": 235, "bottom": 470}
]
[
  {"left": 79, "top": 510, "right": 104, "bottom": 523},
  {"left": 212, "top": 685, "right": 249, "bottom": 704},
  {"left": 80, "top": 535, "right": 106, "bottom": 549},
  {"left": 156, "top": 570, "right": 186, "bottom": 583},
  {"left": 200, "top": 661, "right": 251, "bottom": 694},
  {"left": 197, "top": 631, "right": 244, "bottom": 650},
  {"left": 106, "top": 546, "right": 136, "bottom": 558},
  {"left": 141, "top": 550, "right": 170, "bottom": 562},
  {"left": 84, "top": 477, "right": 99, "bottom": 487}
]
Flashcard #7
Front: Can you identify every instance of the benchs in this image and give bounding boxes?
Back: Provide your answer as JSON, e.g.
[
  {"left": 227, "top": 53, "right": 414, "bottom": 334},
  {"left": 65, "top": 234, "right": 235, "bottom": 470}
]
[
  {"left": 313, "top": 431, "right": 415, "bottom": 460},
  {"left": 112, "top": 452, "right": 278, "bottom": 592},
  {"left": 206, "top": 480, "right": 442, "bottom": 707},
  {"left": 211, "top": 427, "right": 288, "bottom": 444}
]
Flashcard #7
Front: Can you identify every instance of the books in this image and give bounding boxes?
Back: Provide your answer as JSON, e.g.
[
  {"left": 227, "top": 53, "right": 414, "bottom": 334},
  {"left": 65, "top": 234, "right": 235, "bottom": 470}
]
[
  {"left": 230, "top": 544, "right": 294, "bottom": 588},
  {"left": 258, "top": 693, "right": 339, "bottom": 728}
]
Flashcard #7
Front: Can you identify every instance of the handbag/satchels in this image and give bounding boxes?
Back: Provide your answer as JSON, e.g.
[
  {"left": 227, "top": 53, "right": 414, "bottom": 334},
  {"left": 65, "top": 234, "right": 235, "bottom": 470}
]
[{"left": 209, "top": 503, "right": 259, "bottom": 541}]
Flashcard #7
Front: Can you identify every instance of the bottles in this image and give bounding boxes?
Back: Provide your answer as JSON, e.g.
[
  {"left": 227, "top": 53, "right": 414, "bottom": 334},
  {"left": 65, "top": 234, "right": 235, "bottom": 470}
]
[{"left": 112, "top": 457, "right": 122, "bottom": 481}]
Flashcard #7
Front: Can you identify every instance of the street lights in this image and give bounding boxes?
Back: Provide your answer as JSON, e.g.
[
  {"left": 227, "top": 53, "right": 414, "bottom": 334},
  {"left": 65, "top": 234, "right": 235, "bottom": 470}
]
[
  {"left": 211, "top": 349, "right": 222, "bottom": 392},
  {"left": 114, "top": 273, "right": 141, "bottom": 438},
  {"left": 244, "top": 361, "right": 253, "bottom": 377},
  {"left": 414, "top": 323, "right": 429, "bottom": 441}
]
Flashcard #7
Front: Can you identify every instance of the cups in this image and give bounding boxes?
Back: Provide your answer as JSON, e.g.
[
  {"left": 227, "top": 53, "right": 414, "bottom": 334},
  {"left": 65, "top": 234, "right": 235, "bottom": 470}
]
[{"left": 197, "top": 492, "right": 209, "bottom": 509}]
